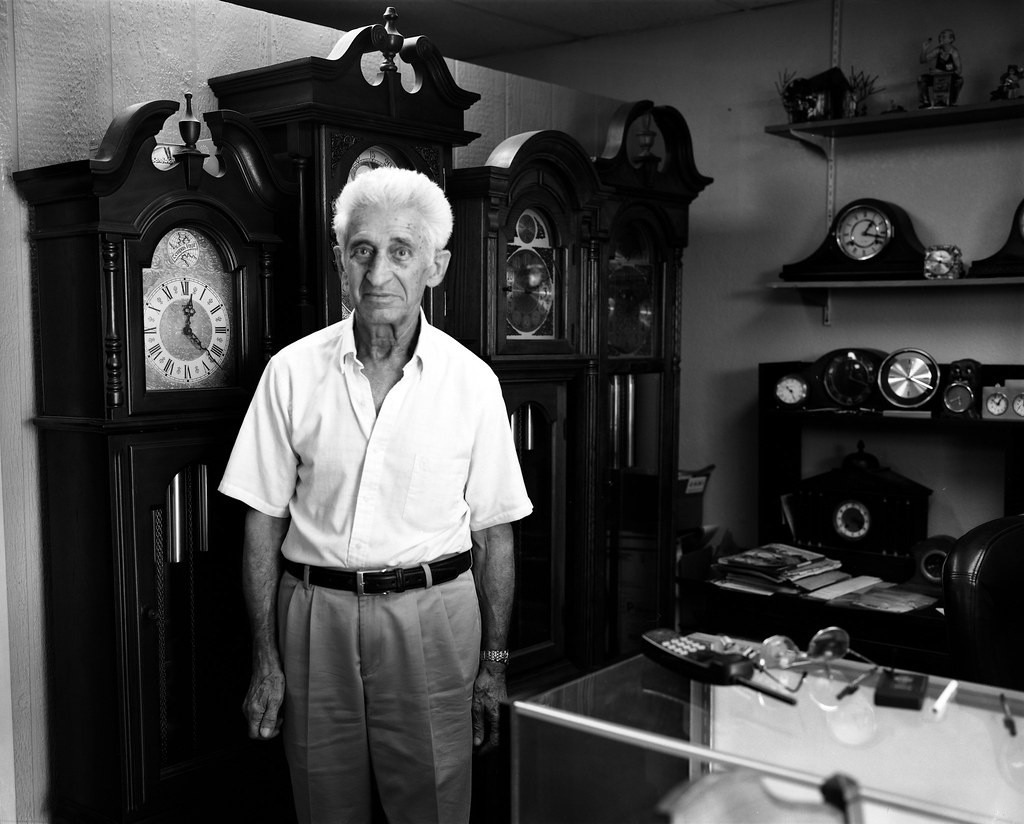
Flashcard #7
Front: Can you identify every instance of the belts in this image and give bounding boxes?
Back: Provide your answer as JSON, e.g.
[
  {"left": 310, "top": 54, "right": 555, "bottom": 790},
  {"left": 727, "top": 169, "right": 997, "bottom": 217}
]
[{"left": 285, "top": 551, "right": 472, "bottom": 595}]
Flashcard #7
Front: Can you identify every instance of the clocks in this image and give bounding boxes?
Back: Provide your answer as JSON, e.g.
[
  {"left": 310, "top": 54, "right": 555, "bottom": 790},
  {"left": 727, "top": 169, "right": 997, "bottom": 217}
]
[
  {"left": 944, "top": 384, "right": 973, "bottom": 412},
  {"left": 774, "top": 375, "right": 808, "bottom": 403},
  {"left": 982, "top": 387, "right": 1024, "bottom": 420},
  {"left": 795, "top": 440, "right": 933, "bottom": 584},
  {"left": 782, "top": 198, "right": 929, "bottom": 283},
  {"left": 901, "top": 535, "right": 959, "bottom": 595},
  {"left": 877, "top": 348, "right": 940, "bottom": 409},
  {"left": 920, "top": 245, "right": 961, "bottom": 281},
  {"left": 823, "top": 356, "right": 878, "bottom": 407},
  {"left": 7, "top": 7, "right": 715, "bottom": 824}
]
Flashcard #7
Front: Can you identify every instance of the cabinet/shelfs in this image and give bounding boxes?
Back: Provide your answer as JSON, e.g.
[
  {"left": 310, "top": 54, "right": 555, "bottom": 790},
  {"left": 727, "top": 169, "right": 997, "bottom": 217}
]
[
  {"left": 513, "top": 630, "right": 1022, "bottom": 824},
  {"left": 762, "top": 97, "right": 1024, "bottom": 556}
]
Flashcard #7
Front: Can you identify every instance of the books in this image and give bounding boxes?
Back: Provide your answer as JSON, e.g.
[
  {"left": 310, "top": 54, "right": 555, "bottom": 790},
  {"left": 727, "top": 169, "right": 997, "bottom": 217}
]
[{"left": 712, "top": 543, "right": 849, "bottom": 597}]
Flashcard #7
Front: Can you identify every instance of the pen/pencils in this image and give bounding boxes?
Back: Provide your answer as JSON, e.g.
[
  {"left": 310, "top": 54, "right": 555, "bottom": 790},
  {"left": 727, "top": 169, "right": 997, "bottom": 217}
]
[
  {"left": 836, "top": 668, "right": 877, "bottom": 701},
  {"left": 1000, "top": 695, "right": 1017, "bottom": 737}
]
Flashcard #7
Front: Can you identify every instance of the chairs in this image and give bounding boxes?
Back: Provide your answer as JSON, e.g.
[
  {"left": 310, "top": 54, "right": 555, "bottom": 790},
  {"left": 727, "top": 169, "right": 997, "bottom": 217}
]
[{"left": 943, "top": 513, "right": 1024, "bottom": 690}]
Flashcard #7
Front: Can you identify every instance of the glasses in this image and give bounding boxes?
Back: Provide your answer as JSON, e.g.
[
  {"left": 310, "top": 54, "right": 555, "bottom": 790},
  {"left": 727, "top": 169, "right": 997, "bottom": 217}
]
[{"left": 749, "top": 627, "right": 877, "bottom": 691}]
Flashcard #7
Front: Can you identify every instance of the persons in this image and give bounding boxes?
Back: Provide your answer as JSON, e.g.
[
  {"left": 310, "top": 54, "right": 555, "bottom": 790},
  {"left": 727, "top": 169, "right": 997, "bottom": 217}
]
[
  {"left": 914, "top": 29, "right": 964, "bottom": 108},
  {"left": 215, "top": 167, "right": 535, "bottom": 824}
]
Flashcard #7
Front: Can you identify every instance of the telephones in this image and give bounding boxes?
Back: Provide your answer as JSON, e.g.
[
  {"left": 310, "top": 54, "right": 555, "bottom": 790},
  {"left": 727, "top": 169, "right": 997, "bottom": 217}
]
[{"left": 641, "top": 625, "right": 798, "bottom": 706}]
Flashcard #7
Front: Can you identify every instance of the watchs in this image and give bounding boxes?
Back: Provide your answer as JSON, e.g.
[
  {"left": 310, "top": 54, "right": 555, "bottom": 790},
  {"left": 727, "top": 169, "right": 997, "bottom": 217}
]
[{"left": 480, "top": 646, "right": 510, "bottom": 667}]
